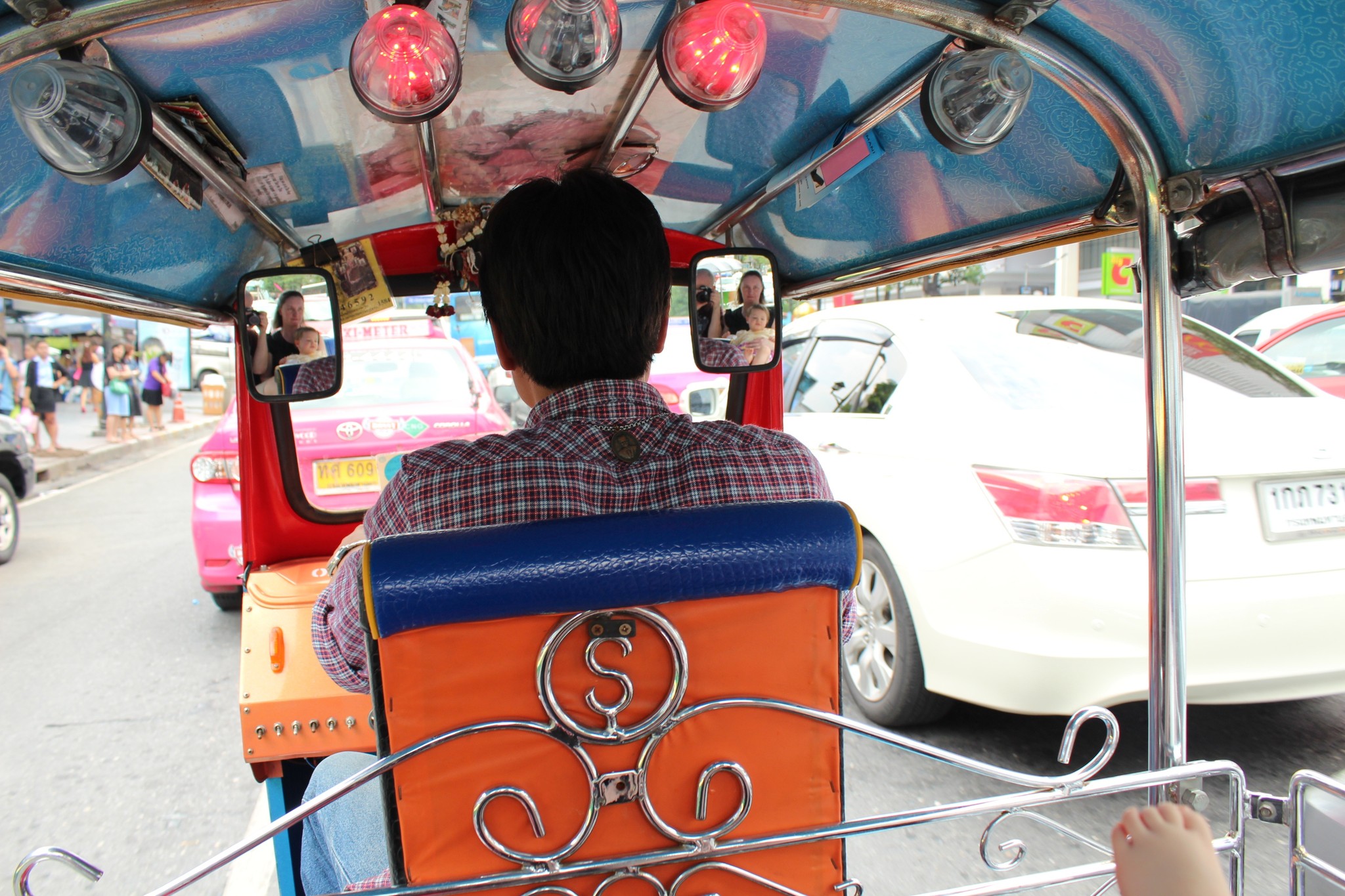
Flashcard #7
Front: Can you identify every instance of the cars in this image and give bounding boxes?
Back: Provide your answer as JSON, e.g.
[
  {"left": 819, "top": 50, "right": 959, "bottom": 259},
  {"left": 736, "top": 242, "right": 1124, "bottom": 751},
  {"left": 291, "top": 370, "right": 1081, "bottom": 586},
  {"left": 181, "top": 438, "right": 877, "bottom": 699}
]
[
  {"left": 190, "top": 315, "right": 521, "bottom": 613},
  {"left": 396, "top": 292, "right": 723, "bottom": 422},
  {"left": 188, "top": 321, "right": 237, "bottom": 388},
  {"left": 675, "top": 291, "right": 1345, "bottom": 736}
]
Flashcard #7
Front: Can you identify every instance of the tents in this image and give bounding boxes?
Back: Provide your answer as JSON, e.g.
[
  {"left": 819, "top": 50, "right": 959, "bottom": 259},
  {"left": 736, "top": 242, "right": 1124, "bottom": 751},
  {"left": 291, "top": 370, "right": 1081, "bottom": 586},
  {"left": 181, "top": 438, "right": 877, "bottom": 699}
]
[{"left": 24, "top": 314, "right": 136, "bottom": 334}]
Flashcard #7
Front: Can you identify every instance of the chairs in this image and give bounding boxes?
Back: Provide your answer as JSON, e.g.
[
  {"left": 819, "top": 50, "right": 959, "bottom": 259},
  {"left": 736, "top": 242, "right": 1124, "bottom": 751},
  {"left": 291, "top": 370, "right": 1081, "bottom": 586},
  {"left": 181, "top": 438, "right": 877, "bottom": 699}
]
[
  {"left": 353, "top": 496, "right": 861, "bottom": 896},
  {"left": 274, "top": 362, "right": 303, "bottom": 394}
]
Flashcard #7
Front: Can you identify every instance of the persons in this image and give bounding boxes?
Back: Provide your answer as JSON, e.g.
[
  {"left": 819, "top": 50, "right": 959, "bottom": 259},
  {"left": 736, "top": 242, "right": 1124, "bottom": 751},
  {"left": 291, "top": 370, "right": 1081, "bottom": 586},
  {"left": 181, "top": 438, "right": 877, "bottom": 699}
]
[
  {"left": 294, "top": 163, "right": 873, "bottom": 896},
  {"left": 103, "top": 342, "right": 174, "bottom": 443},
  {"left": 1111, "top": 801, "right": 1235, "bottom": 894},
  {"left": 696, "top": 256, "right": 776, "bottom": 367},
  {"left": 245, "top": 289, "right": 336, "bottom": 390},
  {"left": 0, "top": 336, "right": 64, "bottom": 452},
  {"left": 56, "top": 340, "right": 107, "bottom": 413}
]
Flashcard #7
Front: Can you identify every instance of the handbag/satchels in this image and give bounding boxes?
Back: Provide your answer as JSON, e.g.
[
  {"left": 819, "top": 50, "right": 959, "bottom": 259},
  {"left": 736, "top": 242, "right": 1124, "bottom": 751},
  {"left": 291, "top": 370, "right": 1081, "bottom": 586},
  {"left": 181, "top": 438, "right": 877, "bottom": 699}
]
[
  {"left": 162, "top": 381, "right": 177, "bottom": 399},
  {"left": 108, "top": 377, "right": 129, "bottom": 394},
  {"left": 16, "top": 407, "right": 38, "bottom": 435}
]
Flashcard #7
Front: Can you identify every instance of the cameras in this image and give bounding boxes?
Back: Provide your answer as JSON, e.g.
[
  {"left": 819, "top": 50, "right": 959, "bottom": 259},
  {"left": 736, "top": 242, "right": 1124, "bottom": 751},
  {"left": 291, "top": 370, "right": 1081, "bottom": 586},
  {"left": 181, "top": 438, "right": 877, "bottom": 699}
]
[
  {"left": 244, "top": 309, "right": 260, "bottom": 327},
  {"left": 696, "top": 285, "right": 713, "bottom": 303}
]
